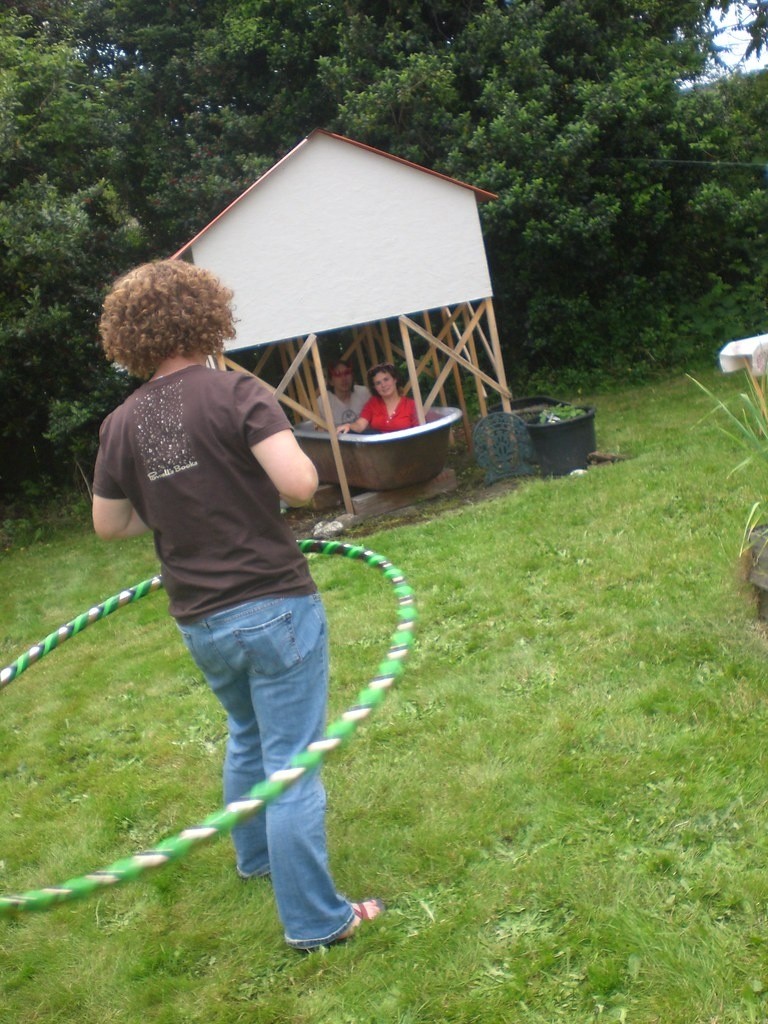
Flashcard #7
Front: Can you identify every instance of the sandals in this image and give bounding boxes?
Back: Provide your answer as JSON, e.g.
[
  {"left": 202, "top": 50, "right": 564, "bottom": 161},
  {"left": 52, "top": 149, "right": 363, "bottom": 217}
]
[{"left": 306, "top": 898, "right": 386, "bottom": 951}]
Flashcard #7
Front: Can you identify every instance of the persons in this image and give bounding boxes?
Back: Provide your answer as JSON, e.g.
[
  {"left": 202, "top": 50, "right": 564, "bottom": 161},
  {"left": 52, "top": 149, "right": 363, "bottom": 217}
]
[
  {"left": 314, "top": 361, "right": 371, "bottom": 432},
  {"left": 92, "top": 259, "right": 384, "bottom": 950},
  {"left": 337, "top": 363, "right": 418, "bottom": 435}
]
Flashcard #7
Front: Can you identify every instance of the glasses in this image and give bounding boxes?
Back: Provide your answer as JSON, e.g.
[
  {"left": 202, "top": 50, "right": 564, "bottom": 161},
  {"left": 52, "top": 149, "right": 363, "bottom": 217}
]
[
  {"left": 331, "top": 368, "right": 352, "bottom": 377},
  {"left": 367, "top": 361, "right": 394, "bottom": 376}
]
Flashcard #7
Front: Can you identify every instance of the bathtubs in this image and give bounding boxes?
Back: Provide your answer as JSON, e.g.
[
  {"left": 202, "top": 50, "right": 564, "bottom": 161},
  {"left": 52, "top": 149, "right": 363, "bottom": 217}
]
[{"left": 292, "top": 407, "right": 462, "bottom": 490}]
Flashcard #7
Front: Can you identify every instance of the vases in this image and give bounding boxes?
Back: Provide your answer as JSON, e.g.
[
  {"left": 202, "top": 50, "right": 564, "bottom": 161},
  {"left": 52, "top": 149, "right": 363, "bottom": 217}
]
[{"left": 488, "top": 396, "right": 571, "bottom": 415}]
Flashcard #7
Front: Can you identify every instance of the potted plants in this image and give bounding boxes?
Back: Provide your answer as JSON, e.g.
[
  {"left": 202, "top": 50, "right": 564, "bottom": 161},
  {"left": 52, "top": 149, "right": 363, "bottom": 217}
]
[{"left": 528, "top": 402, "right": 597, "bottom": 477}]
